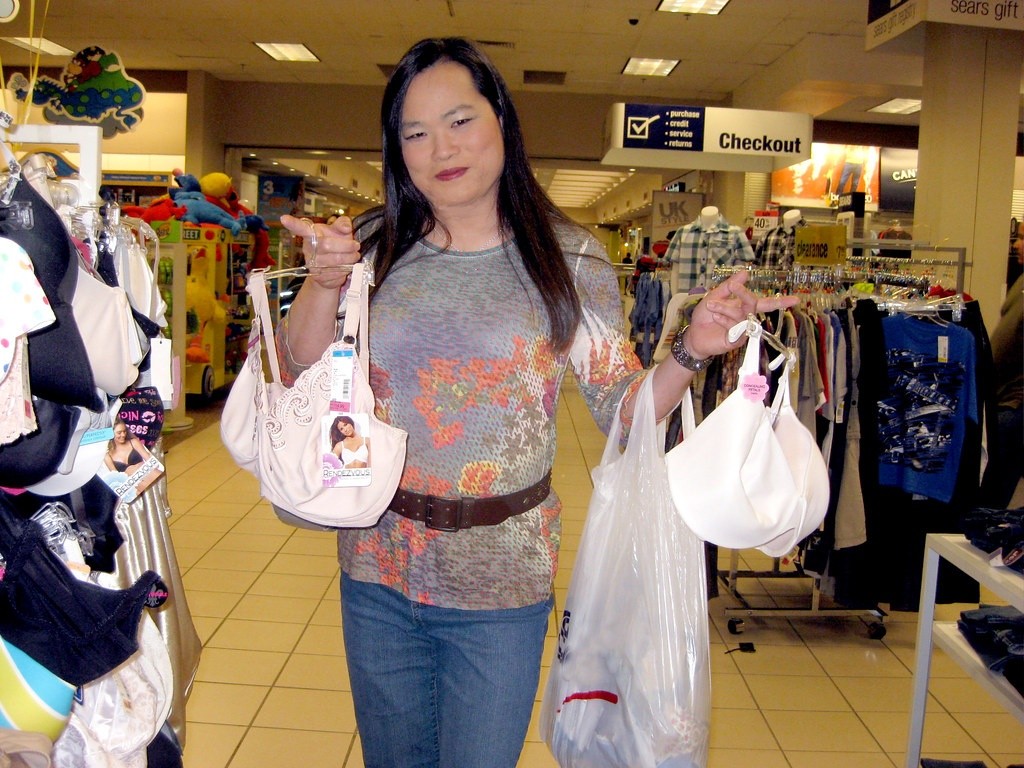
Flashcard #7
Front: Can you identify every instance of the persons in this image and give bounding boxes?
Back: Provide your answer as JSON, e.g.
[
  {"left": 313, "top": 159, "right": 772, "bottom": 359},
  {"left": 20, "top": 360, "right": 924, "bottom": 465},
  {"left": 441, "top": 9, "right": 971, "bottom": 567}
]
[
  {"left": 830, "top": 145, "right": 866, "bottom": 206},
  {"left": 329, "top": 417, "right": 371, "bottom": 468},
  {"left": 275, "top": 38, "right": 803, "bottom": 768},
  {"left": 665, "top": 206, "right": 755, "bottom": 300},
  {"left": 623, "top": 252, "right": 632, "bottom": 294},
  {"left": 974, "top": 269, "right": 1024, "bottom": 508},
  {"left": 754, "top": 209, "right": 810, "bottom": 269},
  {"left": 104, "top": 419, "right": 163, "bottom": 495}
]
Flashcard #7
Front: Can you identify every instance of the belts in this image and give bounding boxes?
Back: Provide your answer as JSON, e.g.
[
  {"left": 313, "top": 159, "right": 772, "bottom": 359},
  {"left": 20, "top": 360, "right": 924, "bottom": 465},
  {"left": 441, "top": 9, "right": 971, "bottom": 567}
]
[{"left": 386, "top": 465, "right": 553, "bottom": 531}]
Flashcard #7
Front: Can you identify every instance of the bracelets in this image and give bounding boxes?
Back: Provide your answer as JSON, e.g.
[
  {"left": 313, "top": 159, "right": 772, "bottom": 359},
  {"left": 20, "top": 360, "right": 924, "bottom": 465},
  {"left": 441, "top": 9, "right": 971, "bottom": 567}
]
[{"left": 670, "top": 324, "right": 714, "bottom": 372}]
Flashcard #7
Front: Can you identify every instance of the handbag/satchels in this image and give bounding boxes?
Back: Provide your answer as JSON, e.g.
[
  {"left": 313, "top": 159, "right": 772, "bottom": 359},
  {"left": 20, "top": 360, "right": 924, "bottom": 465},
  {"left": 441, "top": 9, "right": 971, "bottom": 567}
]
[{"left": 539, "top": 364, "right": 713, "bottom": 768}]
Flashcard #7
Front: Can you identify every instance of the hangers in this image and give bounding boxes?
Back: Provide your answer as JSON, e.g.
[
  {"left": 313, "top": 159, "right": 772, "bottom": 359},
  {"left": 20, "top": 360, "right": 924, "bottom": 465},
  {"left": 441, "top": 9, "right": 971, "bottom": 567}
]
[
  {"left": 245, "top": 217, "right": 378, "bottom": 291},
  {"left": 1, "top": 146, "right": 161, "bottom": 262},
  {"left": 644, "top": 252, "right": 973, "bottom": 375}
]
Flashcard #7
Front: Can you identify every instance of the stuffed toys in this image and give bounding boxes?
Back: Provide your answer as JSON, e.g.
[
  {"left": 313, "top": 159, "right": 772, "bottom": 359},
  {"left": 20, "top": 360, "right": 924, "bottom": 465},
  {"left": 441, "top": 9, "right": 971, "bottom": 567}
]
[{"left": 125, "top": 171, "right": 273, "bottom": 360}]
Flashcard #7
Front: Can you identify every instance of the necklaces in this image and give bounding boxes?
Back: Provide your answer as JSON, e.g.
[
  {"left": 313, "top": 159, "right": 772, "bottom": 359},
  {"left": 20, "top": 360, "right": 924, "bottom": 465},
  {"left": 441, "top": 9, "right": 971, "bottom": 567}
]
[{"left": 427, "top": 218, "right": 502, "bottom": 272}]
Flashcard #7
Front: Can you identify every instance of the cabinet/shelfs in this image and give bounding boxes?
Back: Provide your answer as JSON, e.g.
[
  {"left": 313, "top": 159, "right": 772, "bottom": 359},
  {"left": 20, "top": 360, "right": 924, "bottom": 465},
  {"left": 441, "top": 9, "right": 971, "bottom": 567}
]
[{"left": 905, "top": 531, "right": 1024, "bottom": 768}]
